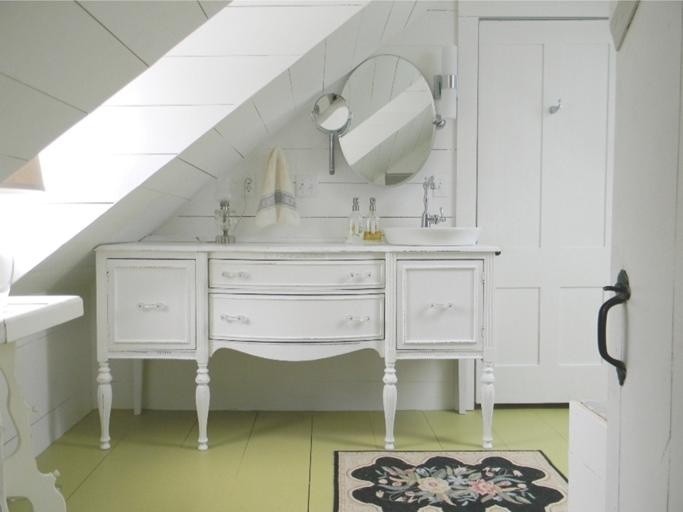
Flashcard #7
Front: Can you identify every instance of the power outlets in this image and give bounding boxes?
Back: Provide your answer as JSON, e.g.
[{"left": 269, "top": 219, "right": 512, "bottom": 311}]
[{"left": 241, "top": 175, "right": 256, "bottom": 198}]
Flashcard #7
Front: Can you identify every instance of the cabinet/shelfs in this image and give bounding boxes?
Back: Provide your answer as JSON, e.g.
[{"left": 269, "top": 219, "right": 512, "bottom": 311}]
[
  {"left": 208, "top": 260, "right": 385, "bottom": 342},
  {"left": 397, "top": 259, "right": 485, "bottom": 351},
  {"left": 108, "top": 259, "right": 197, "bottom": 350},
  {"left": 567, "top": 399, "right": 607, "bottom": 512}
]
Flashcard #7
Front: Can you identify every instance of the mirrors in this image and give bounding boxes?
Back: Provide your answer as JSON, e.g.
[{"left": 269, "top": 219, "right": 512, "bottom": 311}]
[
  {"left": 337, "top": 54, "right": 436, "bottom": 188},
  {"left": 312, "top": 93, "right": 350, "bottom": 135}
]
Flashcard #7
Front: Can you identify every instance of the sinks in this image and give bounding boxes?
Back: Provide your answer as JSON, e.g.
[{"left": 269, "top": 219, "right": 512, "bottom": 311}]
[{"left": 383, "top": 225, "right": 479, "bottom": 246}]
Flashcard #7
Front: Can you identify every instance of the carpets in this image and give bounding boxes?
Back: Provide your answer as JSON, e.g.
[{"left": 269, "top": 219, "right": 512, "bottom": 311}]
[{"left": 334, "top": 449, "right": 568, "bottom": 511}]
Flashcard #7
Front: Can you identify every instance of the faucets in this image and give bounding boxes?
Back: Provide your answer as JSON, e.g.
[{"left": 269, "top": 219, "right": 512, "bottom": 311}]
[{"left": 421, "top": 174, "right": 446, "bottom": 227}]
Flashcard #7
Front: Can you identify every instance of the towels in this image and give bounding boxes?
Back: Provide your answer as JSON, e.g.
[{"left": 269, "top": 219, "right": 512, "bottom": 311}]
[{"left": 256, "top": 145, "right": 300, "bottom": 227}]
